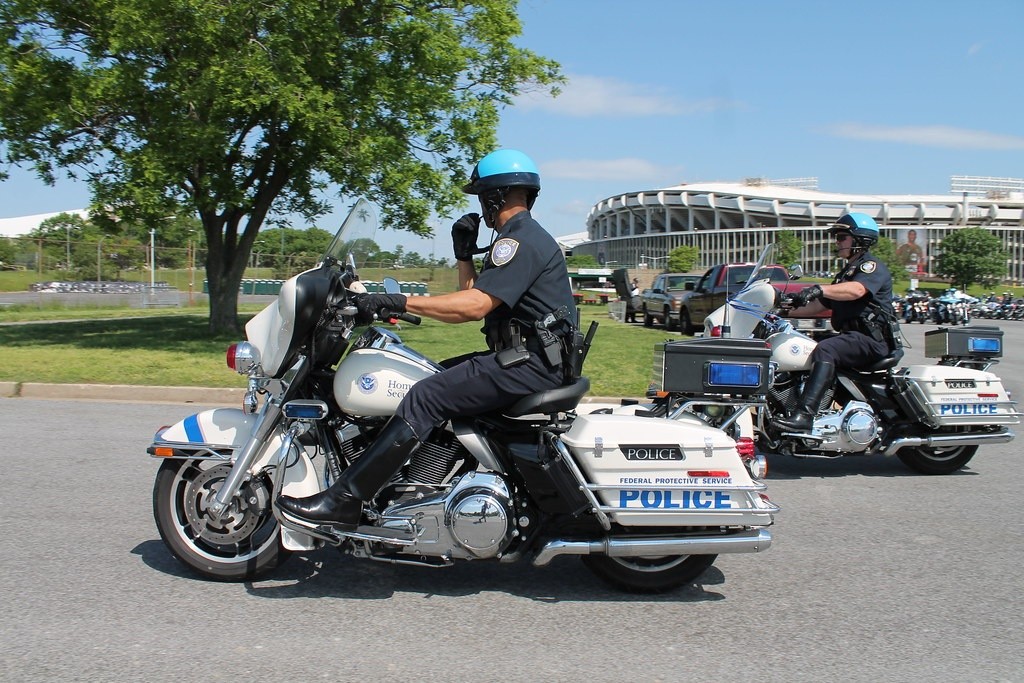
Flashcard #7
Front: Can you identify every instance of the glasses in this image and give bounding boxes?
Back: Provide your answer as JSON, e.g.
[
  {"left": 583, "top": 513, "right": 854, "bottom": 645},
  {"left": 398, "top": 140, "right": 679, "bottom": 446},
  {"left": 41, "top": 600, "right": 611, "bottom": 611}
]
[{"left": 836, "top": 234, "right": 849, "bottom": 242}]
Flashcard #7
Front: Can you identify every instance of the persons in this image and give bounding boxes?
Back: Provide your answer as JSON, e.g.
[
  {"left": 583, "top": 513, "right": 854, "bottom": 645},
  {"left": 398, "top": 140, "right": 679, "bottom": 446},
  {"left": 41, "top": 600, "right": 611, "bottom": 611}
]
[
  {"left": 274, "top": 148, "right": 577, "bottom": 533},
  {"left": 625, "top": 278, "right": 640, "bottom": 323},
  {"left": 768, "top": 213, "right": 893, "bottom": 434},
  {"left": 893, "top": 288, "right": 960, "bottom": 320},
  {"left": 895, "top": 230, "right": 924, "bottom": 275},
  {"left": 971, "top": 292, "right": 1024, "bottom": 320}
]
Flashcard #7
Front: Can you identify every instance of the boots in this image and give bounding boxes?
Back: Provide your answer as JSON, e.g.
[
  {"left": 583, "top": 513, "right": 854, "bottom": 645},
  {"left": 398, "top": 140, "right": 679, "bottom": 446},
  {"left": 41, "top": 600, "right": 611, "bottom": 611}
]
[
  {"left": 274, "top": 413, "right": 423, "bottom": 533},
  {"left": 769, "top": 361, "right": 836, "bottom": 435}
]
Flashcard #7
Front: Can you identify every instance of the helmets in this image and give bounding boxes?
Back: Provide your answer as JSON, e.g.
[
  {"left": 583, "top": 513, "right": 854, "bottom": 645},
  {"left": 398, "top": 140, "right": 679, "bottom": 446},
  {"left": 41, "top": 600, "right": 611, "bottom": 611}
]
[
  {"left": 462, "top": 149, "right": 541, "bottom": 197},
  {"left": 909, "top": 286, "right": 1015, "bottom": 300},
  {"left": 633, "top": 278, "right": 639, "bottom": 283},
  {"left": 827, "top": 211, "right": 878, "bottom": 246}
]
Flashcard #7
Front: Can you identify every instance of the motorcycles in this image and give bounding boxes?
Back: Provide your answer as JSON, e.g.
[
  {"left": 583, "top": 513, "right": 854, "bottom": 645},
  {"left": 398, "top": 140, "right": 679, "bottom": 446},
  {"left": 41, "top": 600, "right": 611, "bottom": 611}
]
[
  {"left": 148, "top": 198, "right": 780, "bottom": 594},
  {"left": 707, "top": 239, "right": 1021, "bottom": 474},
  {"left": 896, "top": 288, "right": 1022, "bottom": 322}
]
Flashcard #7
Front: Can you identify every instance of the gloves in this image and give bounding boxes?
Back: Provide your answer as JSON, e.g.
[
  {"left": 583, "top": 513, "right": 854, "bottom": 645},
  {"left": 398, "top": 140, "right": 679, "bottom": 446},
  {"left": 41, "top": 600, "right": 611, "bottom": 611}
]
[
  {"left": 452, "top": 213, "right": 481, "bottom": 260},
  {"left": 787, "top": 284, "right": 823, "bottom": 310},
  {"left": 355, "top": 293, "right": 408, "bottom": 326}
]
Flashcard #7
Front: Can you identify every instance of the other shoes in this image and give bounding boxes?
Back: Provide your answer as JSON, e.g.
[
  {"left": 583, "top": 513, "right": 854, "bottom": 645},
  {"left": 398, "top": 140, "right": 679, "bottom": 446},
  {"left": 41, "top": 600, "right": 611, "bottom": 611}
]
[
  {"left": 625, "top": 320, "right": 632, "bottom": 323},
  {"left": 632, "top": 319, "right": 638, "bottom": 323}
]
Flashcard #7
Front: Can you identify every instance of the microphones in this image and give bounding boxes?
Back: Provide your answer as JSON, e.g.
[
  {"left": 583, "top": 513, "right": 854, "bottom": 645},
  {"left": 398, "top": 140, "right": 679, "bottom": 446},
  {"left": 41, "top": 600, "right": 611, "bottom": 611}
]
[
  {"left": 833, "top": 247, "right": 862, "bottom": 253},
  {"left": 475, "top": 215, "right": 484, "bottom": 223}
]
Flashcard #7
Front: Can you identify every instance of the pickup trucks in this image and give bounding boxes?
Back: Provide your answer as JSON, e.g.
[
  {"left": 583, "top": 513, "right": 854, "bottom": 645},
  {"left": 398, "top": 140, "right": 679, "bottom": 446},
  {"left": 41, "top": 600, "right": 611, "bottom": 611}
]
[{"left": 682, "top": 264, "right": 834, "bottom": 336}]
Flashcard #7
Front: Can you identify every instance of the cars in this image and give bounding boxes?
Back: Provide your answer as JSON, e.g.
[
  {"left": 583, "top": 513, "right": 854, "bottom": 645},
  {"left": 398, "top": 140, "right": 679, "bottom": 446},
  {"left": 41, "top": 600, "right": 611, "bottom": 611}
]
[{"left": 644, "top": 266, "right": 684, "bottom": 330}]
